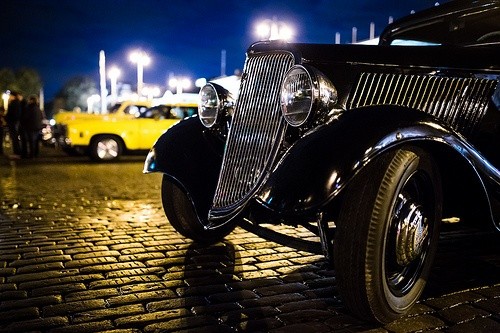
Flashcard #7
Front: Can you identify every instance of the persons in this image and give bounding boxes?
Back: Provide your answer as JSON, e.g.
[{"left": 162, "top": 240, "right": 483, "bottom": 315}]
[
  {"left": 23, "top": 95, "right": 43, "bottom": 163},
  {"left": 4, "top": 91, "right": 20, "bottom": 155},
  {"left": 16, "top": 92, "right": 31, "bottom": 158}
]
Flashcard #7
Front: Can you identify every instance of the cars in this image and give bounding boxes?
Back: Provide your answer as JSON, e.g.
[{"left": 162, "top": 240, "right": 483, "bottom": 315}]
[
  {"left": 142, "top": 0, "right": 500, "bottom": 326},
  {"left": 47, "top": 100, "right": 199, "bottom": 162}
]
